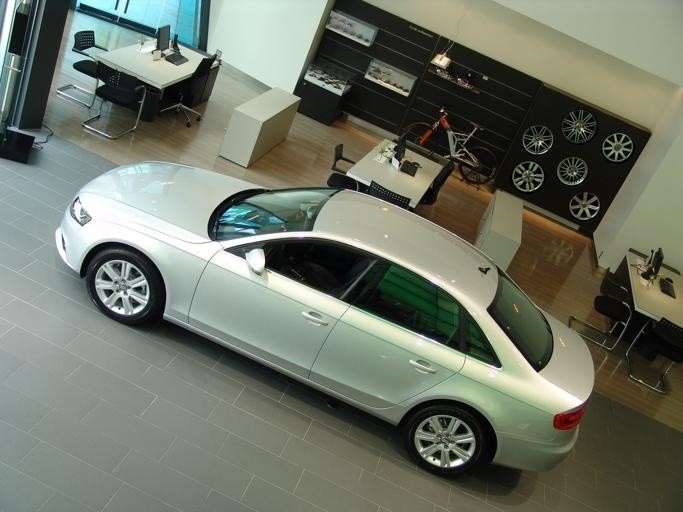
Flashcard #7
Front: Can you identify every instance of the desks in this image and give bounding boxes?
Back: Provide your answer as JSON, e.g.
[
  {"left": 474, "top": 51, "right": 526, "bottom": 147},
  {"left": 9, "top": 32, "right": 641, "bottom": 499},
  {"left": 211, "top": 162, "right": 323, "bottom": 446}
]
[
  {"left": 95, "top": 38, "right": 221, "bottom": 114},
  {"left": 610, "top": 252, "right": 683, "bottom": 360}
]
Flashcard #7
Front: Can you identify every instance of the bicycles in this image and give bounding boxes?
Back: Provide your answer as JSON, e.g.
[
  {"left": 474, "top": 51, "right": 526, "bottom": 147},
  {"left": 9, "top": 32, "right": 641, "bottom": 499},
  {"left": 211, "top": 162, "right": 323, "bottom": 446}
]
[{"left": 401, "top": 103, "right": 497, "bottom": 186}]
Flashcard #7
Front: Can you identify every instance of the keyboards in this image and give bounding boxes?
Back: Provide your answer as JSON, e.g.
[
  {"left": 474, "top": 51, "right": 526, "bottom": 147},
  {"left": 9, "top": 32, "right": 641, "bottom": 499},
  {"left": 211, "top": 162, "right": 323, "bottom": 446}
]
[
  {"left": 401, "top": 159, "right": 418, "bottom": 177},
  {"left": 165, "top": 52, "right": 189, "bottom": 66},
  {"left": 661, "top": 277, "right": 676, "bottom": 297}
]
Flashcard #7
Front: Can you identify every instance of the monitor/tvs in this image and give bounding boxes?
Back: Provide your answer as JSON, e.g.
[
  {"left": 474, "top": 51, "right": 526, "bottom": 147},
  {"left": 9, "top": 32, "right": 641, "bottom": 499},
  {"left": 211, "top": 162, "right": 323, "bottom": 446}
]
[
  {"left": 389, "top": 134, "right": 407, "bottom": 166},
  {"left": 152, "top": 24, "right": 171, "bottom": 58},
  {"left": 640, "top": 247, "right": 664, "bottom": 280}
]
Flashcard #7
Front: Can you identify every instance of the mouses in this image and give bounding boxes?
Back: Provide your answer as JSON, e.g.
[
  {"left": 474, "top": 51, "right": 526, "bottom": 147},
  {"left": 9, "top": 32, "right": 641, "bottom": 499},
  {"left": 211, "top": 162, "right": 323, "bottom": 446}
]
[
  {"left": 411, "top": 161, "right": 420, "bottom": 167},
  {"left": 665, "top": 278, "right": 674, "bottom": 283}
]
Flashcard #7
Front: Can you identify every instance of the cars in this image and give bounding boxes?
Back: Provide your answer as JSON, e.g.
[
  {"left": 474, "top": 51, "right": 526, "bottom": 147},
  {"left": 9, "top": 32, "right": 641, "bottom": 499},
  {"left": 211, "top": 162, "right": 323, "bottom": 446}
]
[{"left": 53, "top": 159, "right": 598, "bottom": 479}]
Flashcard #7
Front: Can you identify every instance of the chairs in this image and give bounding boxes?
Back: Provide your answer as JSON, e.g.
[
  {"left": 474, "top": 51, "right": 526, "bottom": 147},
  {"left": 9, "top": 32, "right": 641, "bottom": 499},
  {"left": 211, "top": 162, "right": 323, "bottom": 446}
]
[
  {"left": 625, "top": 316, "right": 683, "bottom": 394},
  {"left": 326, "top": 129, "right": 454, "bottom": 211},
  {"left": 566, "top": 272, "right": 633, "bottom": 352},
  {"left": 58, "top": 31, "right": 217, "bottom": 139}
]
